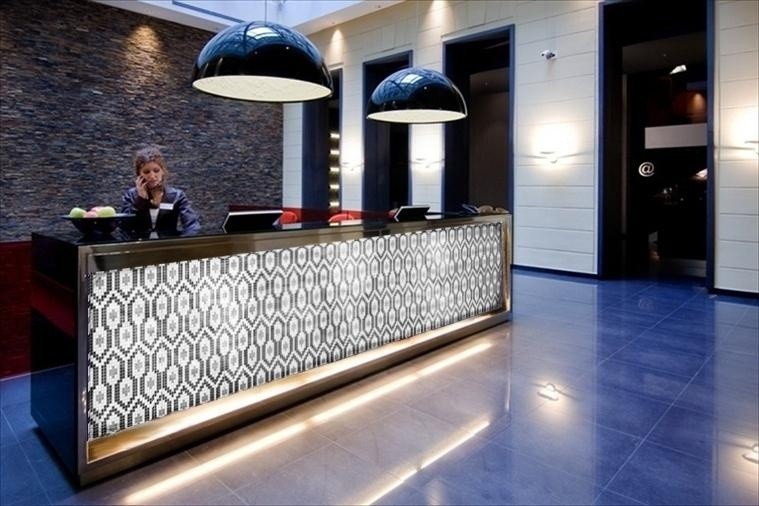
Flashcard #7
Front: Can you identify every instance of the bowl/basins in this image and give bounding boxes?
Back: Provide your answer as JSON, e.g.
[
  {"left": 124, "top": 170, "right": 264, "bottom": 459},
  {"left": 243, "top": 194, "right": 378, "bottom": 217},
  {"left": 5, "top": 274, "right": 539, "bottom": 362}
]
[{"left": 60, "top": 213, "right": 137, "bottom": 234}]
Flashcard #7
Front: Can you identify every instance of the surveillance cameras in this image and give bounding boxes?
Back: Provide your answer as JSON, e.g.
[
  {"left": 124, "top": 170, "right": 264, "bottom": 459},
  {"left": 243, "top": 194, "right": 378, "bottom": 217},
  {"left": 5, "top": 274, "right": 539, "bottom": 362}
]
[{"left": 540, "top": 50, "right": 554, "bottom": 59}]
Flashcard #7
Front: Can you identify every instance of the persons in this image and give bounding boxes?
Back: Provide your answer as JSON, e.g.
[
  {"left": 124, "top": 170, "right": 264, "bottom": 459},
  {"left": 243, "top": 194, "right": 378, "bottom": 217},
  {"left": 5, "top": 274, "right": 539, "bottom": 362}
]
[{"left": 115, "top": 149, "right": 201, "bottom": 241}]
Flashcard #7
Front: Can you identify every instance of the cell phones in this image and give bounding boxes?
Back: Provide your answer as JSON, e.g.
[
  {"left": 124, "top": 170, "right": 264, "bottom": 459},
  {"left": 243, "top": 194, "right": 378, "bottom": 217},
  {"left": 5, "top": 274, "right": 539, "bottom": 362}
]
[{"left": 138, "top": 176, "right": 149, "bottom": 190}]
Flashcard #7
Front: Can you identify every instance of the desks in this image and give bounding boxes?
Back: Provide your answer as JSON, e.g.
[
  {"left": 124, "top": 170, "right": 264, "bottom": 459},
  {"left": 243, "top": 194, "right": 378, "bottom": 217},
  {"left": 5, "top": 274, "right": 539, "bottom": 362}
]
[{"left": 30, "top": 211, "right": 512, "bottom": 486}]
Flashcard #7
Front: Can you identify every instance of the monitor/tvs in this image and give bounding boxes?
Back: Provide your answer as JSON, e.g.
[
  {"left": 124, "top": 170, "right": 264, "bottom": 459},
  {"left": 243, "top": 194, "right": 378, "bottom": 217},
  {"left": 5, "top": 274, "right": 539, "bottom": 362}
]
[
  {"left": 393, "top": 206, "right": 430, "bottom": 222},
  {"left": 220, "top": 210, "right": 283, "bottom": 229}
]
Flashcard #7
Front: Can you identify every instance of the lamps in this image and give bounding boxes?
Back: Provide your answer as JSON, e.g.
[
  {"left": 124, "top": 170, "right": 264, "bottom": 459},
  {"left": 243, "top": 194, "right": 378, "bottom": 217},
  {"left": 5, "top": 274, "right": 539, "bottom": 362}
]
[
  {"left": 365, "top": 67, "right": 468, "bottom": 123},
  {"left": 191, "top": 21, "right": 334, "bottom": 104}
]
